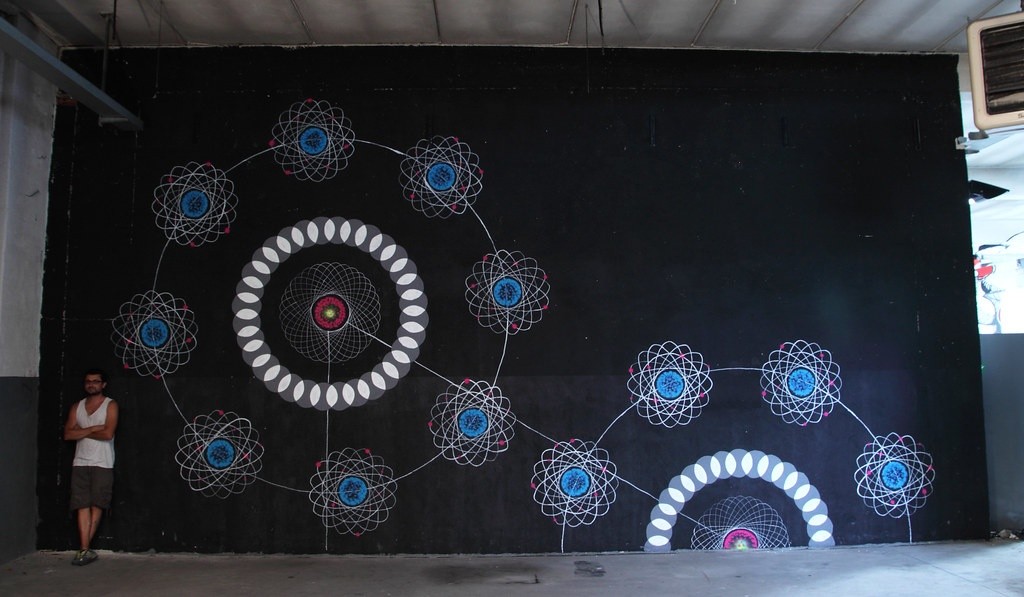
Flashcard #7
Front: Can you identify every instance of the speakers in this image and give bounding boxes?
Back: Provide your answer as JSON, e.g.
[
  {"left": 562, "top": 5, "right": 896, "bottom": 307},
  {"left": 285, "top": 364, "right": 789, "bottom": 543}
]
[{"left": 967, "top": 10, "right": 1024, "bottom": 130}]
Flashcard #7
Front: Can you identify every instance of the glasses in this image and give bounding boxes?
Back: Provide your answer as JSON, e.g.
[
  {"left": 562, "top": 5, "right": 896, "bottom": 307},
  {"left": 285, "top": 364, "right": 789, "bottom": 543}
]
[{"left": 85, "top": 379, "right": 101, "bottom": 384}]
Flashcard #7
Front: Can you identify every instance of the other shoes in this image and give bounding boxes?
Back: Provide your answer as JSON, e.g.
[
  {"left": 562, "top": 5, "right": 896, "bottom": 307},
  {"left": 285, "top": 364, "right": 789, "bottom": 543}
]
[{"left": 71, "top": 549, "right": 98, "bottom": 565}]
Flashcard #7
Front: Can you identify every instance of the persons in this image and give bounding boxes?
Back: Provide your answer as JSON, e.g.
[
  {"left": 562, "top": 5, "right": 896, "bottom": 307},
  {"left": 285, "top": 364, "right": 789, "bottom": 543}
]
[{"left": 63, "top": 368, "right": 119, "bottom": 566}]
